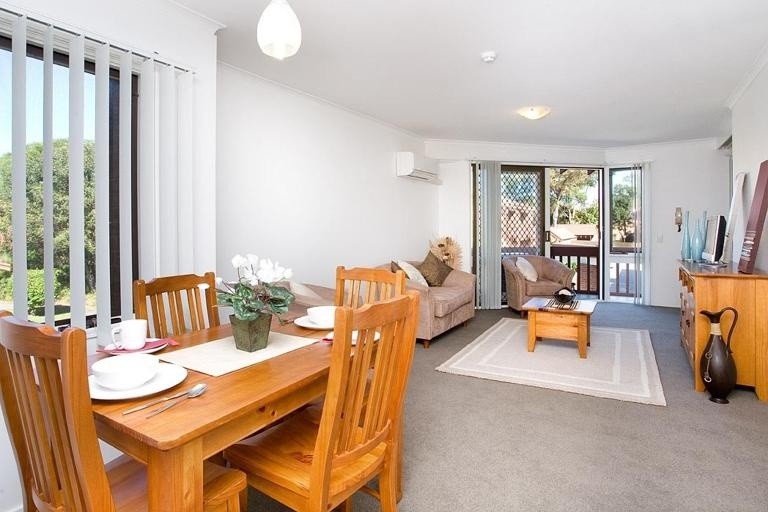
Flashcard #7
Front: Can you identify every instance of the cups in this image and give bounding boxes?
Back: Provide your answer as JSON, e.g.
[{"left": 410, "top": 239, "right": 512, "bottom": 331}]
[{"left": 112, "top": 318, "right": 147, "bottom": 350}]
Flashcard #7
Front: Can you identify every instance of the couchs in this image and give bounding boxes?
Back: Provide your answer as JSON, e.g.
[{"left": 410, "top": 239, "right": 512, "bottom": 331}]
[
  {"left": 500, "top": 254, "right": 575, "bottom": 317},
  {"left": 367, "top": 261, "right": 476, "bottom": 349}
]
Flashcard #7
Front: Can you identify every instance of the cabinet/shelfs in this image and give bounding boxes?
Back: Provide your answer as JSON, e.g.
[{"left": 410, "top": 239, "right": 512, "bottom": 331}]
[{"left": 678, "top": 259, "right": 768, "bottom": 402}]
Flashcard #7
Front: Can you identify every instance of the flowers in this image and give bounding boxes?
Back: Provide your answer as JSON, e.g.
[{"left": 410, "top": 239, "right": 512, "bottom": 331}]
[
  {"left": 427, "top": 233, "right": 462, "bottom": 268},
  {"left": 195, "top": 252, "right": 301, "bottom": 326}
]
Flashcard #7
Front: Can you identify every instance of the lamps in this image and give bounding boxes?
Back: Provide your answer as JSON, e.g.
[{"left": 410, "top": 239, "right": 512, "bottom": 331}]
[
  {"left": 257, "top": 0, "right": 302, "bottom": 60},
  {"left": 675, "top": 207, "right": 683, "bottom": 232}
]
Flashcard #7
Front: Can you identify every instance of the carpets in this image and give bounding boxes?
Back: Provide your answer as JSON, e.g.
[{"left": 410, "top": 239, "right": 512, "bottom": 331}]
[{"left": 434, "top": 316, "right": 666, "bottom": 407}]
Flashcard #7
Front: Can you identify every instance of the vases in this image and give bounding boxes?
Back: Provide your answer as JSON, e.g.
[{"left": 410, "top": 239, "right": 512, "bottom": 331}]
[
  {"left": 698, "top": 306, "right": 739, "bottom": 404},
  {"left": 680, "top": 208, "right": 709, "bottom": 263},
  {"left": 229, "top": 313, "right": 272, "bottom": 353}
]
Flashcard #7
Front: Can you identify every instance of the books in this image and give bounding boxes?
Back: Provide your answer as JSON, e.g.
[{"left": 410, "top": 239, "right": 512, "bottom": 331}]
[{"left": 698, "top": 261, "right": 728, "bottom": 268}]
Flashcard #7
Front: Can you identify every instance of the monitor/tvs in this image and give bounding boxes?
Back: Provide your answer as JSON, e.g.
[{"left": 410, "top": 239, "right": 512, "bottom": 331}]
[{"left": 701, "top": 215, "right": 726, "bottom": 265}]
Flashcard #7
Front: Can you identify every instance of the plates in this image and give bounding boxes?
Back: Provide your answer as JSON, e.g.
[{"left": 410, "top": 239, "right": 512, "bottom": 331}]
[
  {"left": 325, "top": 329, "right": 382, "bottom": 346},
  {"left": 87, "top": 364, "right": 187, "bottom": 399},
  {"left": 105, "top": 338, "right": 168, "bottom": 356},
  {"left": 293, "top": 316, "right": 334, "bottom": 330}
]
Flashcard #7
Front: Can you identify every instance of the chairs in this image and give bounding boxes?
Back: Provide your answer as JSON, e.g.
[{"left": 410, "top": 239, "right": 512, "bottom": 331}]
[
  {"left": 0, "top": 308, "right": 247, "bottom": 512},
  {"left": 133, "top": 271, "right": 222, "bottom": 338},
  {"left": 222, "top": 289, "right": 421, "bottom": 510},
  {"left": 334, "top": 264, "right": 404, "bottom": 314}
]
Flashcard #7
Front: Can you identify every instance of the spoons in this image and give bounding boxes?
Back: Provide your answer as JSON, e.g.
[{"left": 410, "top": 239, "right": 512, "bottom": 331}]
[{"left": 140, "top": 381, "right": 209, "bottom": 419}]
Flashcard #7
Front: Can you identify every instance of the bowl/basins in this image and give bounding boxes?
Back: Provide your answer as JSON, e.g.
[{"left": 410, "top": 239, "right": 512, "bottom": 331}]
[
  {"left": 306, "top": 306, "right": 338, "bottom": 324},
  {"left": 554, "top": 286, "right": 576, "bottom": 302},
  {"left": 92, "top": 353, "right": 159, "bottom": 389}
]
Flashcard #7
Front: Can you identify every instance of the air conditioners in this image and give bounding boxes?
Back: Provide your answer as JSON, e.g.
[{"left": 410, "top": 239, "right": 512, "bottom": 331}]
[{"left": 395, "top": 150, "right": 442, "bottom": 180}]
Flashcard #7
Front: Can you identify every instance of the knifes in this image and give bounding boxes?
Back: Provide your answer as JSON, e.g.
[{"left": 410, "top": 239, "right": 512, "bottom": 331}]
[{"left": 123, "top": 384, "right": 196, "bottom": 414}]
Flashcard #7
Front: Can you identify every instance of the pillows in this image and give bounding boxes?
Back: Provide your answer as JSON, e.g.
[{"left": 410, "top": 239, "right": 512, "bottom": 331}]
[
  {"left": 383, "top": 251, "right": 453, "bottom": 287},
  {"left": 513, "top": 256, "right": 538, "bottom": 282}
]
